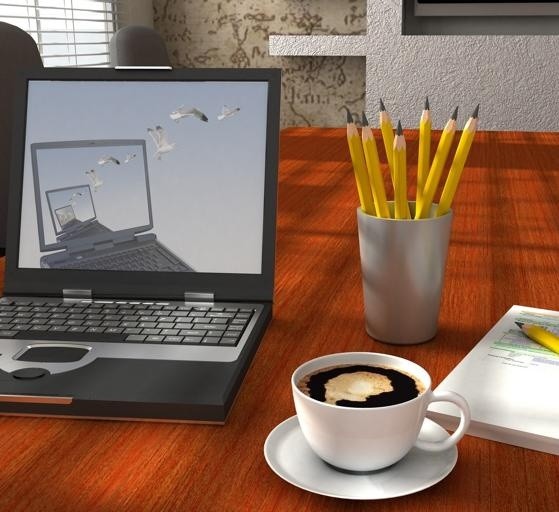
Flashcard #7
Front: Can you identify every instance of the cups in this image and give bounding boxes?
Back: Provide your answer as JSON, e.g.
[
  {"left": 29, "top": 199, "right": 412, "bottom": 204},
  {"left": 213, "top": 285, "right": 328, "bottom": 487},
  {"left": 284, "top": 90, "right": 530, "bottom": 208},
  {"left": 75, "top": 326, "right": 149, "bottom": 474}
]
[
  {"left": 291, "top": 352, "right": 471, "bottom": 474},
  {"left": 357, "top": 201, "right": 452, "bottom": 344}
]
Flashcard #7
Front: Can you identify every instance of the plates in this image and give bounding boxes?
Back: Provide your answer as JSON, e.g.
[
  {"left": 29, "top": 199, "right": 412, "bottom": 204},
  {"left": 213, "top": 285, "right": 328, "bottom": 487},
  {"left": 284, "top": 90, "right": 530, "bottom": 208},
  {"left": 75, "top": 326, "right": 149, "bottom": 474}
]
[{"left": 264, "top": 412, "right": 459, "bottom": 501}]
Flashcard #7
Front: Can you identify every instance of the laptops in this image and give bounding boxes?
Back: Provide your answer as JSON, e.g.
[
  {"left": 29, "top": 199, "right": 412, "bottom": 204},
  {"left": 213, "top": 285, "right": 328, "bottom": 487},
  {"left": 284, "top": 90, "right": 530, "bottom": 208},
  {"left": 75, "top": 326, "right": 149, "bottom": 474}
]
[{"left": 0, "top": 66, "right": 281, "bottom": 420}]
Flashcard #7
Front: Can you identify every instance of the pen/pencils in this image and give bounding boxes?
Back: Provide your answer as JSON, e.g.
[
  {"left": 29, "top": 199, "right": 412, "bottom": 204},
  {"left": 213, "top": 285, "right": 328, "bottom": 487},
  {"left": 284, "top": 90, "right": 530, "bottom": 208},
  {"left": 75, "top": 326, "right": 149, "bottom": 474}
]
[
  {"left": 346, "top": 96, "right": 480, "bottom": 220},
  {"left": 515, "top": 322, "right": 559, "bottom": 355}
]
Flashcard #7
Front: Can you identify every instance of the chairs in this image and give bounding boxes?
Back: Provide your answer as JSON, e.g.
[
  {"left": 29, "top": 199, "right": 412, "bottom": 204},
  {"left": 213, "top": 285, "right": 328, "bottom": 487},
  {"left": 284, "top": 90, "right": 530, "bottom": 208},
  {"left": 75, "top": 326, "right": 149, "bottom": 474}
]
[{"left": 108, "top": 25, "right": 170, "bottom": 68}]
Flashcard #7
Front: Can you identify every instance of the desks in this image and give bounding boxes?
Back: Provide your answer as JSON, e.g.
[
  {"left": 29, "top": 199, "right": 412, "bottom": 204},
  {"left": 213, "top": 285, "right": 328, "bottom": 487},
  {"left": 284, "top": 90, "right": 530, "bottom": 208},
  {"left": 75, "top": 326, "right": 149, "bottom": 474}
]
[{"left": 1, "top": 125, "right": 559, "bottom": 512}]
